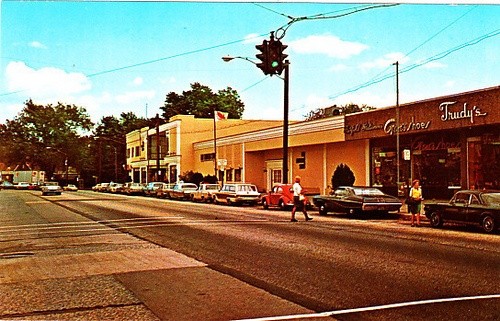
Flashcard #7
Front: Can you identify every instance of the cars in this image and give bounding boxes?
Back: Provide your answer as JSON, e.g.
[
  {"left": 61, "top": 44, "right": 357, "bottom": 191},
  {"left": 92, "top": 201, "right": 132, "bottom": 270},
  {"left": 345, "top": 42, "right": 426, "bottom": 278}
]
[
  {"left": 62, "top": 184, "right": 78, "bottom": 191},
  {"left": 92, "top": 182, "right": 221, "bottom": 204},
  {"left": 214, "top": 183, "right": 262, "bottom": 207},
  {"left": 261, "top": 184, "right": 314, "bottom": 211},
  {"left": 42, "top": 181, "right": 63, "bottom": 195},
  {"left": 0, "top": 181, "right": 43, "bottom": 190},
  {"left": 312, "top": 186, "right": 403, "bottom": 219},
  {"left": 424, "top": 188, "right": 500, "bottom": 234}
]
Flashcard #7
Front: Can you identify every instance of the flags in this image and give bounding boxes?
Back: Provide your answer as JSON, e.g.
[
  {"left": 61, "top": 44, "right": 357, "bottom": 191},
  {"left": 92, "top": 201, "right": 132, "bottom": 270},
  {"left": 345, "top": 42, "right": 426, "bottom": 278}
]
[{"left": 214, "top": 110, "right": 229, "bottom": 121}]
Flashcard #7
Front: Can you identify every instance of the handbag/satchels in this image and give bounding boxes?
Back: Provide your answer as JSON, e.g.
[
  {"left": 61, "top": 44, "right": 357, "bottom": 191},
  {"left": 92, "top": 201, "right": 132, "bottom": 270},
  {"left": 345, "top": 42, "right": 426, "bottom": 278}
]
[
  {"left": 405, "top": 197, "right": 412, "bottom": 203},
  {"left": 299, "top": 194, "right": 305, "bottom": 201}
]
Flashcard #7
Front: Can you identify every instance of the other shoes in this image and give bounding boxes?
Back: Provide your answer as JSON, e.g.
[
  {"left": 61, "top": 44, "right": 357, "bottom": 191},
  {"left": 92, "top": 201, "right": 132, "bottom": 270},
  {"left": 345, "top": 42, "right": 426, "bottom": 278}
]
[
  {"left": 291, "top": 219, "right": 297, "bottom": 222},
  {"left": 416, "top": 223, "right": 422, "bottom": 227},
  {"left": 411, "top": 223, "right": 414, "bottom": 227},
  {"left": 305, "top": 218, "right": 312, "bottom": 221}
]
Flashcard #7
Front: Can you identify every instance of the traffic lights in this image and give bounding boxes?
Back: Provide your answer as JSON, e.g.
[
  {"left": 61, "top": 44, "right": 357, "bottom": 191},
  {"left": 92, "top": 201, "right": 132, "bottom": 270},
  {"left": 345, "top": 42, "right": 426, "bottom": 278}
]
[
  {"left": 269, "top": 40, "right": 281, "bottom": 70},
  {"left": 254, "top": 39, "right": 269, "bottom": 75},
  {"left": 276, "top": 42, "right": 291, "bottom": 75}
]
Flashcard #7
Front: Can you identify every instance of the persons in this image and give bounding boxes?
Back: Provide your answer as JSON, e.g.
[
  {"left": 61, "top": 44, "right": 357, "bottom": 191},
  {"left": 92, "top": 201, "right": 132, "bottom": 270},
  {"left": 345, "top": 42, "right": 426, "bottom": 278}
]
[
  {"left": 290, "top": 176, "right": 313, "bottom": 222},
  {"left": 408, "top": 179, "right": 422, "bottom": 228}
]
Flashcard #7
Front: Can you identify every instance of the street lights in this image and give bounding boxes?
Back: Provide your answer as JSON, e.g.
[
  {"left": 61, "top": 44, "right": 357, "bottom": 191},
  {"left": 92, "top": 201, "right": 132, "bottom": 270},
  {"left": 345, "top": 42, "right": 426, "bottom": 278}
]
[
  {"left": 46, "top": 147, "right": 69, "bottom": 186},
  {"left": 221, "top": 55, "right": 290, "bottom": 184},
  {"left": 87, "top": 144, "right": 102, "bottom": 183},
  {"left": 392, "top": 61, "right": 401, "bottom": 213},
  {"left": 107, "top": 144, "right": 117, "bottom": 182},
  {"left": 93, "top": 136, "right": 128, "bottom": 182}
]
